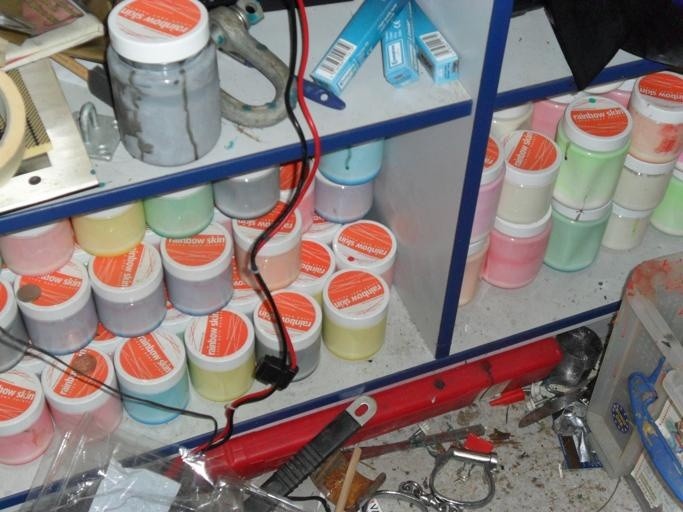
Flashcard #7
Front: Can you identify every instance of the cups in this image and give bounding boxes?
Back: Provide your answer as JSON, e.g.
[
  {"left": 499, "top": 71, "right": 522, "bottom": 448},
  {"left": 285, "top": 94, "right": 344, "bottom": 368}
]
[{"left": 555, "top": 326, "right": 602, "bottom": 388}]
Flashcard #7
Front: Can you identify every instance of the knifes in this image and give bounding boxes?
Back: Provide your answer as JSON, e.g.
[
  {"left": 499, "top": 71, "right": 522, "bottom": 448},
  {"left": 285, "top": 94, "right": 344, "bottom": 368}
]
[{"left": 519, "top": 389, "right": 593, "bottom": 429}]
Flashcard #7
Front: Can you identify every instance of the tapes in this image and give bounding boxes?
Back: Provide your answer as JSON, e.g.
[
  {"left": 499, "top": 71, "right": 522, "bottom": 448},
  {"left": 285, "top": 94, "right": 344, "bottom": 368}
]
[{"left": 0, "top": 72, "right": 25, "bottom": 188}]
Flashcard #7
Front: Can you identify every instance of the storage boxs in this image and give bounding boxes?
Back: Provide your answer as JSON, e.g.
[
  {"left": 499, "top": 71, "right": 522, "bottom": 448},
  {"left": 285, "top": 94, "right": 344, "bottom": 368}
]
[{"left": 586, "top": 257, "right": 681, "bottom": 507}]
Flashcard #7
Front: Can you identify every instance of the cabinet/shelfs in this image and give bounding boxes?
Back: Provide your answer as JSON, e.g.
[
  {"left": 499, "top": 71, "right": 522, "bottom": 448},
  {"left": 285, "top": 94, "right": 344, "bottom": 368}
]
[{"left": 1, "top": 0, "right": 675, "bottom": 506}]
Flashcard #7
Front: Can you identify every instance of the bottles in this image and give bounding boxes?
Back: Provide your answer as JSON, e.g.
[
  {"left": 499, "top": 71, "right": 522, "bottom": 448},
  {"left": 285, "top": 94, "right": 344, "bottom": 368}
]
[
  {"left": 108, "top": 0, "right": 221, "bottom": 167},
  {"left": 456, "top": 68, "right": 683, "bottom": 299},
  {"left": 1, "top": 139, "right": 397, "bottom": 467}
]
